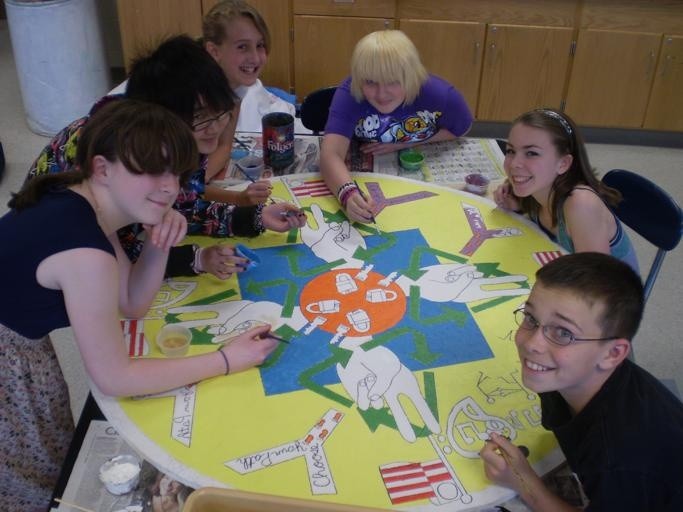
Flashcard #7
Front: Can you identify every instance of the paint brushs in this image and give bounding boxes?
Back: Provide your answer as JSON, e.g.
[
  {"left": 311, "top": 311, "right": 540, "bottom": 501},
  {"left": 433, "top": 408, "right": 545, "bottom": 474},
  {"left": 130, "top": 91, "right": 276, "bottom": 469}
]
[
  {"left": 233, "top": 136, "right": 249, "bottom": 153},
  {"left": 352, "top": 178, "right": 383, "bottom": 239},
  {"left": 498, "top": 447, "right": 531, "bottom": 494},
  {"left": 260, "top": 330, "right": 289, "bottom": 347},
  {"left": 233, "top": 161, "right": 280, "bottom": 206}
]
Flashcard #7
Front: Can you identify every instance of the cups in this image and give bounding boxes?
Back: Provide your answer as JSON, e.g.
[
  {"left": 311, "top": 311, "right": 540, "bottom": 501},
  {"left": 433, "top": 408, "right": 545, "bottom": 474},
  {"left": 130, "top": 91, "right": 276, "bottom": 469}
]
[
  {"left": 399, "top": 151, "right": 426, "bottom": 170},
  {"left": 464, "top": 173, "right": 490, "bottom": 196},
  {"left": 262, "top": 111, "right": 296, "bottom": 170}
]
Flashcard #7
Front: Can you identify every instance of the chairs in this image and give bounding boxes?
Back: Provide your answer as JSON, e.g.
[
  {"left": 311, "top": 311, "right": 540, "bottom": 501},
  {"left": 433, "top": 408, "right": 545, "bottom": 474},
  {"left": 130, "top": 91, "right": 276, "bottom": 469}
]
[{"left": 596, "top": 168, "right": 682, "bottom": 305}]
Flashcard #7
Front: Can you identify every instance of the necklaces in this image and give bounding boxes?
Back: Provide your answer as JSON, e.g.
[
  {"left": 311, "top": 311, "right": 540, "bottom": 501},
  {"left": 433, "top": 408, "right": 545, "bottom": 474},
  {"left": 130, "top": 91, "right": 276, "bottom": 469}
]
[{"left": 85, "top": 181, "right": 108, "bottom": 229}]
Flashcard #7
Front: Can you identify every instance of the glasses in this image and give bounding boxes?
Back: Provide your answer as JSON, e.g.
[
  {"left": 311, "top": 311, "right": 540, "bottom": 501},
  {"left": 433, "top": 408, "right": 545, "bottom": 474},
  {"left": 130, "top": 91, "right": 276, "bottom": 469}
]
[
  {"left": 192, "top": 109, "right": 228, "bottom": 131},
  {"left": 514, "top": 307, "right": 618, "bottom": 346}
]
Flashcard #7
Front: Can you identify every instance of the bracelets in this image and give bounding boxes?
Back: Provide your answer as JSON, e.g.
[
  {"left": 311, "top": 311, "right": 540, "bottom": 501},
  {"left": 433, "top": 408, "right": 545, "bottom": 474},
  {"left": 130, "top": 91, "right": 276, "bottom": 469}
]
[
  {"left": 335, "top": 182, "right": 358, "bottom": 208},
  {"left": 218, "top": 348, "right": 231, "bottom": 376},
  {"left": 193, "top": 248, "right": 203, "bottom": 273}
]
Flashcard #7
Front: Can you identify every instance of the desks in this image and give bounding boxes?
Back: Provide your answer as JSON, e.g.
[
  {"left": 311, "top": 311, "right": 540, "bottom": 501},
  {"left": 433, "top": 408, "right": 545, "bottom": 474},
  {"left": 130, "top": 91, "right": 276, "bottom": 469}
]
[{"left": 44, "top": 137, "right": 591, "bottom": 511}]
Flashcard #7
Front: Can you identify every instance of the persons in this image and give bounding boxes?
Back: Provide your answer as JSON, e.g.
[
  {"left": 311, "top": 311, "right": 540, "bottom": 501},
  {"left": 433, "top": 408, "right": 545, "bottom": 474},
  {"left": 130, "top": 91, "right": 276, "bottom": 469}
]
[
  {"left": 492, "top": 108, "right": 641, "bottom": 278},
  {"left": 321, "top": 28, "right": 474, "bottom": 223},
  {"left": 19, "top": 31, "right": 306, "bottom": 282},
  {"left": 477, "top": 251, "right": 681, "bottom": 511},
  {"left": 104, "top": 0, "right": 270, "bottom": 183},
  {"left": 1, "top": 98, "right": 280, "bottom": 512}
]
